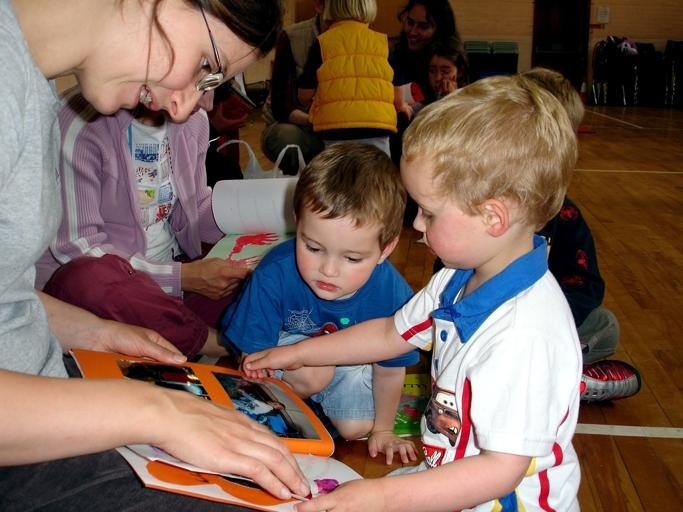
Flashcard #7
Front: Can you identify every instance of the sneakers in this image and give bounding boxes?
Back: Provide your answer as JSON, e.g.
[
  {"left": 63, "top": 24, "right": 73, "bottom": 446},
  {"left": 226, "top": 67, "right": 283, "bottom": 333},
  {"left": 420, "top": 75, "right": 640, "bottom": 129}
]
[{"left": 572, "top": 358, "right": 642, "bottom": 402}]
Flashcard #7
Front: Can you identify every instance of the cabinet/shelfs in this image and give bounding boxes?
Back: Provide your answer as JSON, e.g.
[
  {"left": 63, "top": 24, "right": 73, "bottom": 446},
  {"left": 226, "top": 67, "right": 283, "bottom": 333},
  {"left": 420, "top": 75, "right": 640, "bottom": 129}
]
[{"left": 467, "top": 52, "right": 518, "bottom": 84}]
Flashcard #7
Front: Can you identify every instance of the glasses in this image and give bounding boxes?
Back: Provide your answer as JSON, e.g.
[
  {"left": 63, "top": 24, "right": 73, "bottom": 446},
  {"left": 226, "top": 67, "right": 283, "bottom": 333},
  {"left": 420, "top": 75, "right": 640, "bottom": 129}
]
[{"left": 194, "top": 4, "right": 224, "bottom": 96}]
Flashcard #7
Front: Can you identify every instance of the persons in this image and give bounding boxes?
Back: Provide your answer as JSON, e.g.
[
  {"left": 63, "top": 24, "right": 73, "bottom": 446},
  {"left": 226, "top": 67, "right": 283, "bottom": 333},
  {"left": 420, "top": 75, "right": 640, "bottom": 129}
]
[
  {"left": 238, "top": 67, "right": 586, "bottom": 510},
  {"left": 433, "top": 70, "right": 642, "bottom": 405},
  {"left": 259, "top": 0, "right": 479, "bottom": 176},
  {"left": 0, "top": 0, "right": 320, "bottom": 511},
  {"left": 220, "top": 142, "right": 422, "bottom": 466},
  {"left": 222, "top": 376, "right": 285, "bottom": 418},
  {"left": 35, "top": 83, "right": 249, "bottom": 363}
]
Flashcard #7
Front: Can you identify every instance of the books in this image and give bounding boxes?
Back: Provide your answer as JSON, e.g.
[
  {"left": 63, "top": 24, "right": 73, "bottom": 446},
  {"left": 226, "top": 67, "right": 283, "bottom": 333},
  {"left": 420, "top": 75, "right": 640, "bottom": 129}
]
[{"left": 65, "top": 341, "right": 364, "bottom": 511}]
